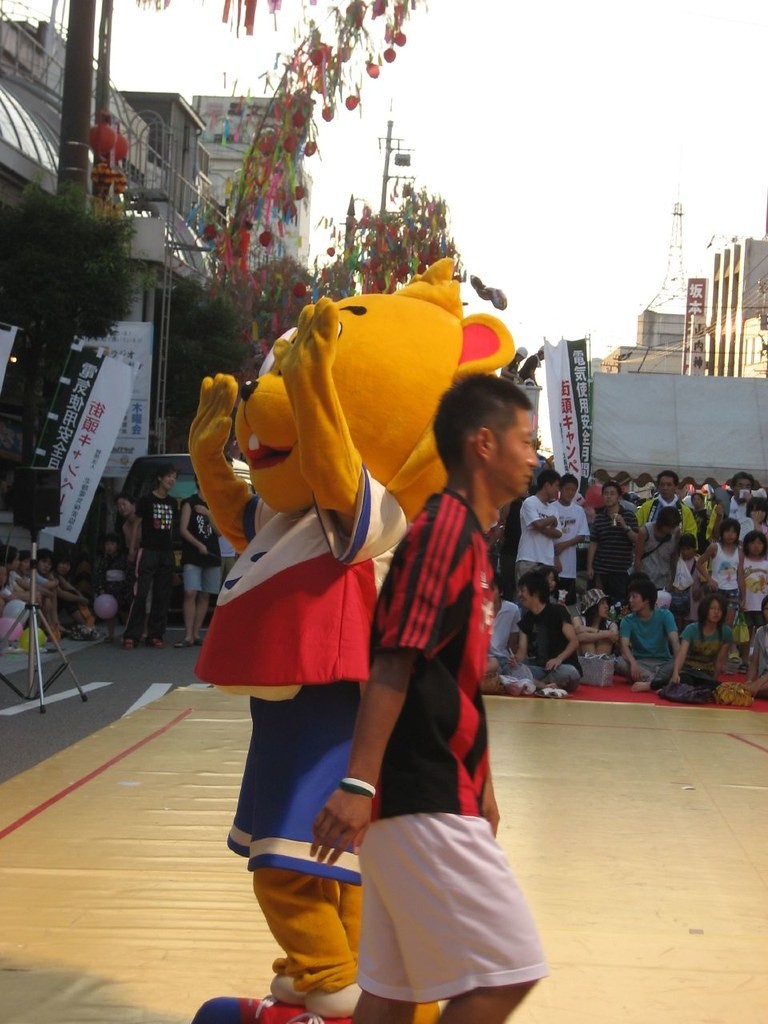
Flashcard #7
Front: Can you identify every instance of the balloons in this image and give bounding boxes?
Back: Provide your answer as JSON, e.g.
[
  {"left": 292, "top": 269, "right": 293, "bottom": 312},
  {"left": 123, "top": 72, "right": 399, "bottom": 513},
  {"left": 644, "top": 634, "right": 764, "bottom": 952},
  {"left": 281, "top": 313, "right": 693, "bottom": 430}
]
[
  {"left": 19, "top": 626, "right": 47, "bottom": 654},
  {"left": 0, "top": 617, "right": 23, "bottom": 643},
  {"left": 94, "top": 593, "right": 118, "bottom": 620},
  {"left": 3, "top": 599, "right": 30, "bottom": 626}
]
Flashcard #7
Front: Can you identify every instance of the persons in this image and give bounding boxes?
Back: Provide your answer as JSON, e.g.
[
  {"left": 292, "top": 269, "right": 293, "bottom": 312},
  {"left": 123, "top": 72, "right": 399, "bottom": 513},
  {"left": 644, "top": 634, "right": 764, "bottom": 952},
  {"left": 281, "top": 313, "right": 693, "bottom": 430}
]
[
  {"left": 0, "top": 465, "right": 245, "bottom": 651},
  {"left": 478, "top": 465, "right": 768, "bottom": 701},
  {"left": 306, "top": 372, "right": 551, "bottom": 1023},
  {"left": 499, "top": 345, "right": 545, "bottom": 387}
]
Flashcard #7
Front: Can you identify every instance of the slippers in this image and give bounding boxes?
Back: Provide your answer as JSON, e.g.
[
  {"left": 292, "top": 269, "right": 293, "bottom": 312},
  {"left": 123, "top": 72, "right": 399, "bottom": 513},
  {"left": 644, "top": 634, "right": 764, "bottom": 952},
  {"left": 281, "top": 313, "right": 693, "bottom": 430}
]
[{"left": 535, "top": 688, "right": 574, "bottom": 698}]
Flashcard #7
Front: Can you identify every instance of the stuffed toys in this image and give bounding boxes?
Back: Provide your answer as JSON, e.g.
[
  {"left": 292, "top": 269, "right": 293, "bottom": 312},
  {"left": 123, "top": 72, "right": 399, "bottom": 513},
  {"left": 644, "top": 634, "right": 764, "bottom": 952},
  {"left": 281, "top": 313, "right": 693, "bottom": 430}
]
[{"left": 185, "top": 254, "right": 518, "bottom": 1023}]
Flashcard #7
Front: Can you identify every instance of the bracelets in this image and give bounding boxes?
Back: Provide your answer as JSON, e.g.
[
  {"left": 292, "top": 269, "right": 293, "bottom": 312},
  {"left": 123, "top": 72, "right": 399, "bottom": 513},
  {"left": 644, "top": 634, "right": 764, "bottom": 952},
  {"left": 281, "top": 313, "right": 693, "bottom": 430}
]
[
  {"left": 338, "top": 775, "right": 377, "bottom": 797},
  {"left": 594, "top": 629, "right": 599, "bottom": 634}
]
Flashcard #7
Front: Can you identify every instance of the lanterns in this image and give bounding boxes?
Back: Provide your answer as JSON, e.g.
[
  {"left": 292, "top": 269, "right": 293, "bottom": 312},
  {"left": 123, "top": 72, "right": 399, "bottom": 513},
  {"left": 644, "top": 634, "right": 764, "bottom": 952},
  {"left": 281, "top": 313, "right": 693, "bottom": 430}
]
[
  {"left": 200, "top": 0, "right": 462, "bottom": 383},
  {"left": 86, "top": 110, "right": 127, "bottom": 201}
]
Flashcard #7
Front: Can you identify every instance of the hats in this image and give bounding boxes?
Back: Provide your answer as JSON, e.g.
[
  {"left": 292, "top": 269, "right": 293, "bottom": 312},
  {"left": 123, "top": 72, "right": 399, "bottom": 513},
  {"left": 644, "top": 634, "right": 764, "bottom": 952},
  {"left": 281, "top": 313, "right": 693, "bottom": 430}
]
[{"left": 582, "top": 589, "right": 608, "bottom": 614}]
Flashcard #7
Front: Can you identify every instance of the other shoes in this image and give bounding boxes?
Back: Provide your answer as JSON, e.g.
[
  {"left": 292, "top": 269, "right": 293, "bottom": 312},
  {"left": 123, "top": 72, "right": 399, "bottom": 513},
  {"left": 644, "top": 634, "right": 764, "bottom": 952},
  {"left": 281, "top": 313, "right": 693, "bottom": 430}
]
[
  {"left": 151, "top": 638, "right": 163, "bottom": 646},
  {"left": 174, "top": 639, "right": 194, "bottom": 647},
  {"left": 728, "top": 653, "right": 740, "bottom": 662},
  {"left": 738, "top": 664, "right": 749, "bottom": 674},
  {"left": 71, "top": 624, "right": 100, "bottom": 641},
  {"left": 123, "top": 639, "right": 133, "bottom": 650}
]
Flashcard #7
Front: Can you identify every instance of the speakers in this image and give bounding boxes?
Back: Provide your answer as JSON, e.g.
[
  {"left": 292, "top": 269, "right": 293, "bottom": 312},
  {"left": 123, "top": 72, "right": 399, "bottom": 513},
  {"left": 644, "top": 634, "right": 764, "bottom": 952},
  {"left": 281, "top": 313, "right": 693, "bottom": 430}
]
[{"left": 13, "top": 465, "right": 61, "bottom": 529}]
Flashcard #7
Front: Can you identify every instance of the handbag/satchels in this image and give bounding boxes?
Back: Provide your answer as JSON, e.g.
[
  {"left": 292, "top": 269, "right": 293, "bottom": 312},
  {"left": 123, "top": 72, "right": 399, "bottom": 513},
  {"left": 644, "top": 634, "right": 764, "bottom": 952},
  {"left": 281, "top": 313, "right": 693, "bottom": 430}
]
[
  {"left": 672, "top": 553, "right": 694, "bottom": 590},
  {"left": 650, "top": 663, "right": 722, "bottom": 704},
  {"left": 712, "top": 682, "right": 753, "bottom": 707},
  {"left": 732, "top": 609, "right": 750, "bottom": 643},
  {"left": 578, "top": 652, "right": 615, "bottom": 687},
  {"left": 670, "top": 594, "right": 691, "bottom": 617}
]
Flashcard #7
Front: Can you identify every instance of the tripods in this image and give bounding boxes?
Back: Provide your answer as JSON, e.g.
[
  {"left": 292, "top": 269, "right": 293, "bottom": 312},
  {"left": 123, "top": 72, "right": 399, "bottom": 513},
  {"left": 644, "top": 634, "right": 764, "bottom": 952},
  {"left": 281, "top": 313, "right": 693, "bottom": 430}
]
[{"left": 0, "top": 528, "right": 89, "bottom": 714}]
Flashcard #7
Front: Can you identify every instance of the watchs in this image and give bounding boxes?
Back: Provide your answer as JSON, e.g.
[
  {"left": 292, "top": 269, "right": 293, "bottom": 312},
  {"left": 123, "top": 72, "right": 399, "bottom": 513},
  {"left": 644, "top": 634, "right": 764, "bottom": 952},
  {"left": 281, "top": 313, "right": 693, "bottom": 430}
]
[{"left": 624, "top": 525, "right": 631, "bottom": 535}]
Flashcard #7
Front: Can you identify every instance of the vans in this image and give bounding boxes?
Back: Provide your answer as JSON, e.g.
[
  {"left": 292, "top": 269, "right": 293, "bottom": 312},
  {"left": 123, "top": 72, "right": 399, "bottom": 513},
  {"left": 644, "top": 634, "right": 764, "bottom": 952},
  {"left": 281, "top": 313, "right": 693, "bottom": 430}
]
[{"left": 107, "top": 454, "right": 253, "bottom": 615}]
